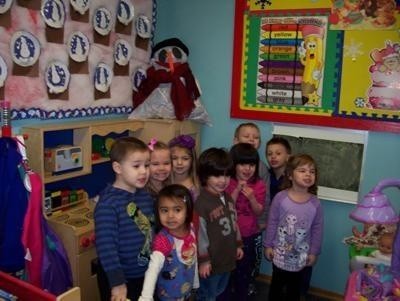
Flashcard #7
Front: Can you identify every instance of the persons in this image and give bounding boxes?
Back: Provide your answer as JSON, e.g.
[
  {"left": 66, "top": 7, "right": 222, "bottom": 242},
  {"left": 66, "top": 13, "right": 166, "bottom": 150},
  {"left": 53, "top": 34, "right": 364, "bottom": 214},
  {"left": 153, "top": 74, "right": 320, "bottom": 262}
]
[
  {"left": 224, "top": 142, "right": 268, "bottom": 301},
  {"left": 166, "top": 135, "right": 201, "bottom": 203},
  {"left": 232, "top": 123, "right": 271, "bottom": 296},
  {"left": 263, "top": 154, "right": 324, "bottom": 301},
  {"left": 94, "top": 136, "right": 159, "bottom": 301},
  {"left": 138, "top": 184, "right": 201, "bottom": 301},
  {"left": 145, "top": 139, "right": 171, "bottom": 199},
  {"left": 264, "top": 136, "right": 319, "bottom": 207},
  {"left": 194, "top": 147, "right": 245, "bottom": 301}
]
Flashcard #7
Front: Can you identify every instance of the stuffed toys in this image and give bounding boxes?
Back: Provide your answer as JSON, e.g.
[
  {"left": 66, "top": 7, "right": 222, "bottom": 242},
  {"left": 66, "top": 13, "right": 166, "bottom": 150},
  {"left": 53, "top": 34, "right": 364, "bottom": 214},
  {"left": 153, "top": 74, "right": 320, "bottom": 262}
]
[{"left": 128, "top": 38, "right": 213, "bottom": 128}]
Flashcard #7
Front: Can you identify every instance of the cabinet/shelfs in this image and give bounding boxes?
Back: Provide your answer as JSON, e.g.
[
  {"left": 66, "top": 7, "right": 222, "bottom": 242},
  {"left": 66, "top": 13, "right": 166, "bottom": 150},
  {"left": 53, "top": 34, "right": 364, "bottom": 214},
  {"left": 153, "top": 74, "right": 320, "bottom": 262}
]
[{"left": 20, "top": 117, "right": 201, "bottom": 301}]
